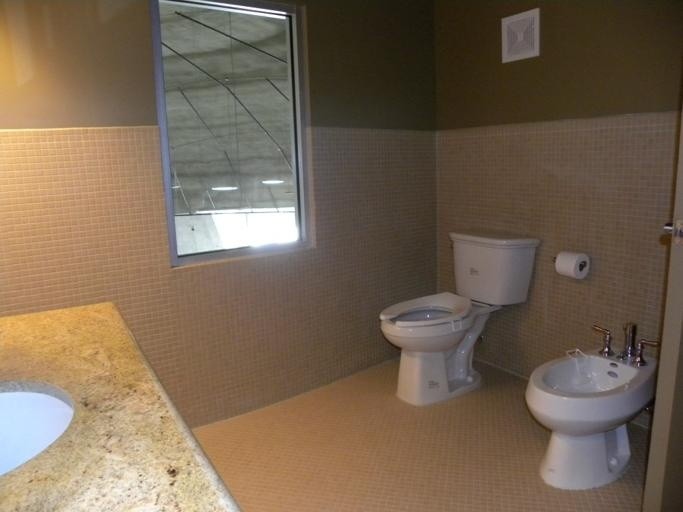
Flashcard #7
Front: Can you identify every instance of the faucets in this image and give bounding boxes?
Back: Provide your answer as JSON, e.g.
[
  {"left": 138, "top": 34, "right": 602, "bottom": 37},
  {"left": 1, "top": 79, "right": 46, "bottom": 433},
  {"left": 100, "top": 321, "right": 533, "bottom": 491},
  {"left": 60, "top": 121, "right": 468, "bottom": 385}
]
[{"left": 621, "top": 320, "right": 637, "bottom": 359}]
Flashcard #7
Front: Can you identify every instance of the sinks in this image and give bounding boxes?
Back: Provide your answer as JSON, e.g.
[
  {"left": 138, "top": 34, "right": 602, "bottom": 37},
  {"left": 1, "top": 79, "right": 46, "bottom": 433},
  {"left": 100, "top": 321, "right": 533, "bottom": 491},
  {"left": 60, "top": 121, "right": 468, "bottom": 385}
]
[
  {"left": 0, "top": 378, "right": 79, "bottom": 480},
  {"left": 524, "top": 345, "right": 658, "bottom": 491}
]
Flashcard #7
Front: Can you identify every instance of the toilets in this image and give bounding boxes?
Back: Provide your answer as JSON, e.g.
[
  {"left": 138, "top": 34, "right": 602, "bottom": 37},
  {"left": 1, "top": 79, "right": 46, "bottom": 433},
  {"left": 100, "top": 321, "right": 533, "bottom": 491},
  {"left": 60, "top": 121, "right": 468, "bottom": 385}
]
[{"left": 378, "top": 229, "right": 539, "bottom": 408}]
